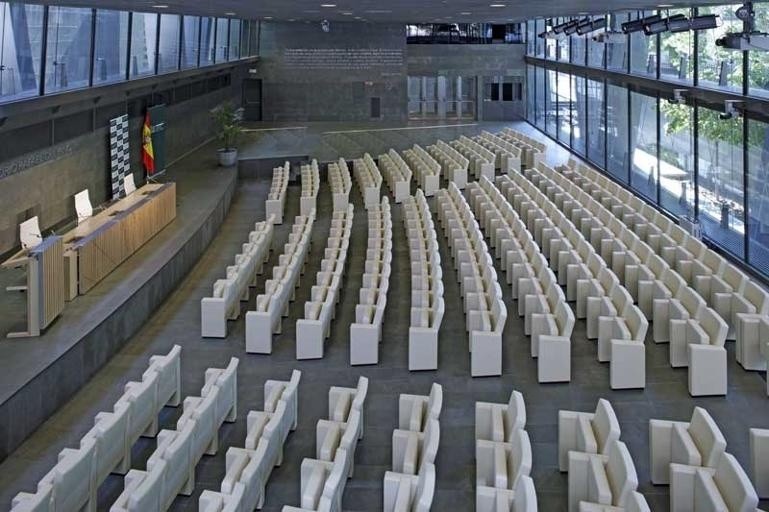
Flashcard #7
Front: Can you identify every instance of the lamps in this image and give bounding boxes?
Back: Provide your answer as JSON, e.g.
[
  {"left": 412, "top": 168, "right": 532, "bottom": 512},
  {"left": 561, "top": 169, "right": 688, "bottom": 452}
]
[{"left": 537, "top": 0, "right": 769, "bottom": 51}]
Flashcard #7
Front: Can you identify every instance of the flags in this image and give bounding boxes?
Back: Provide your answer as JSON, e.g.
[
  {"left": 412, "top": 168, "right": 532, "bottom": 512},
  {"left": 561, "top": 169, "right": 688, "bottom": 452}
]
[{"left": 140, "top": 110, "right": 157, "bottom": 175}]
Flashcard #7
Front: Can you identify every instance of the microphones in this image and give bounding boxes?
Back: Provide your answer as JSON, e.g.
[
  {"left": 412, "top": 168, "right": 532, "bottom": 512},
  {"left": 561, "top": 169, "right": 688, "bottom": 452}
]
[
  {"left": 37, "top": 234, "right": 47, "bottom": 240},
  {"left": 78, "top": 212, "right": 93, "bottom": 228},
  {"left": 24, "top": 243, "right": 30, "bottom": 252}
]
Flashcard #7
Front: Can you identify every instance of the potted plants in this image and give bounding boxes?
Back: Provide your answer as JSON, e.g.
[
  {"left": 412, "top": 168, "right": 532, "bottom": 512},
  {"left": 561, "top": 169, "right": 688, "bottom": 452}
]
[{"left": 206, "top": 96, "right": 244, "bottom": 166}]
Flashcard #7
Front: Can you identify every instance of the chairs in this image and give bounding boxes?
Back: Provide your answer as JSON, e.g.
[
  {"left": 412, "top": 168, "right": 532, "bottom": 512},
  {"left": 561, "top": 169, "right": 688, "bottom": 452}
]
[
  {"left": 124, "top": 173, "right": 137, "bottom": 197},
  {"left": 556, "top": 397, "right": 621, "bottom": 471},
  {"left": 568, "top": 440, "right": 638, "bottom": 509},
  {"left": 475, "top": 474, "right": 537, "bottom": 511},
  {"left": 198, "top": 127, "right": 767, "bottom": 397},
  {"left": 19, "top": 215, "right": 43, "bottom": 252},
  {"left": 649, "top": 404, "right": 728, "bottom": 485},
  {"left": 474, "top": 429, "right": 534, "bottom": 490},
  {"left": 74, "top": 188, "right": 94, "bottom": 226},
  {"left": 2, "top": 344, "right": 444, "bottom": 512},
  {"left": 669, "top": 452, "right": 760, "bottom": 511},
  {"left": 473, "top": 389, "right": 528, "bottom": 441},
  {"left": 578, "top": 489, "right": 652, "bottom": 511},
  {"left": 749, "top": 426, "right": 766, "bottom": 500}
]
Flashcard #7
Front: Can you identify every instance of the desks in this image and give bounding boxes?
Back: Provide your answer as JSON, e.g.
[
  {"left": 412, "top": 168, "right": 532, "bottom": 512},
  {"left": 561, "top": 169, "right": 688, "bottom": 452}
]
[
  {"left": 60, "top": 183, "right": 176, "bottom": 301},
  {"left": 2, "top": 237, "right": 65, "bottom": 339}
]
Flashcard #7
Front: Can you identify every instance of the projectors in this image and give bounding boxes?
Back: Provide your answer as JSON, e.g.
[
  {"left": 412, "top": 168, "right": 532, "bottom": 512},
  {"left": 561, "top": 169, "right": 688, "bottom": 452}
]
[
  {"left": 715, "top": 33, "right": 769, "bottom": 52},
  {"left": 592, "top": 32, "right": 625, "bottom": 43}
]
